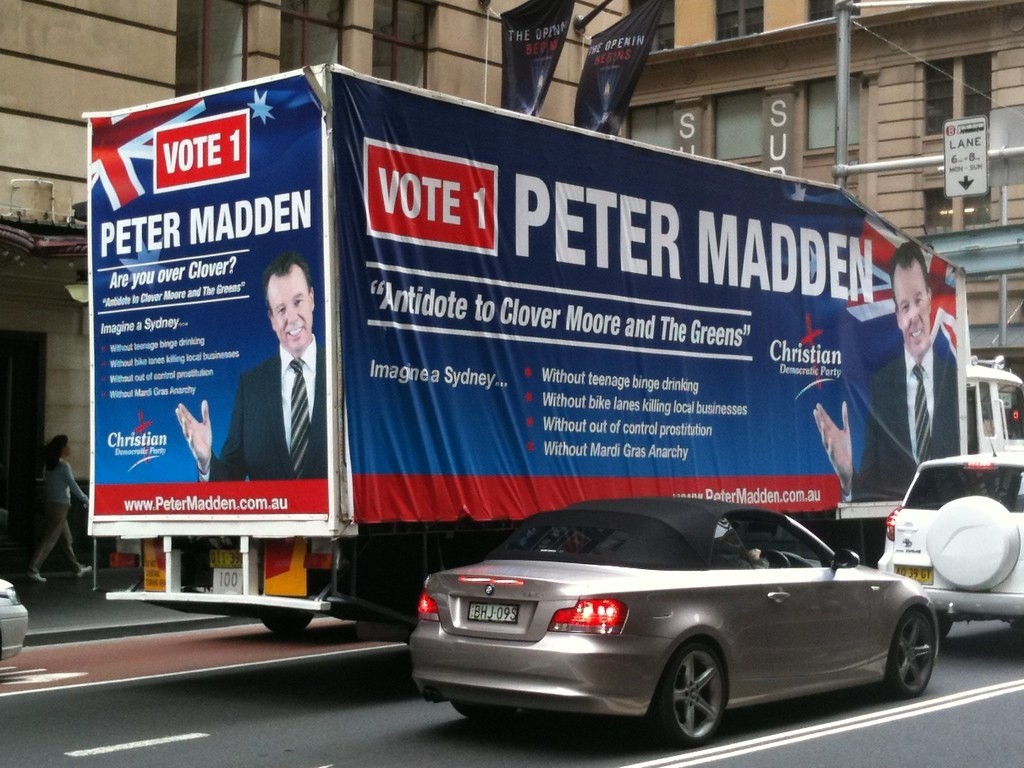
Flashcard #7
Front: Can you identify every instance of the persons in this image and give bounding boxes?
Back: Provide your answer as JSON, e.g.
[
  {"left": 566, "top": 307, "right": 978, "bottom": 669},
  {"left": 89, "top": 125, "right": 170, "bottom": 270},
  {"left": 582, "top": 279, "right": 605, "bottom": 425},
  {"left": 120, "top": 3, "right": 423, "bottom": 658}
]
[
  {"left": 813, "top": 242, "right": 960, "bottom": 502},
  {"left": 174, "top": 252, "right": 326, "bottom": 482},
  {"left": 27, "top": 436, "right": 92, "bottom": 582}
]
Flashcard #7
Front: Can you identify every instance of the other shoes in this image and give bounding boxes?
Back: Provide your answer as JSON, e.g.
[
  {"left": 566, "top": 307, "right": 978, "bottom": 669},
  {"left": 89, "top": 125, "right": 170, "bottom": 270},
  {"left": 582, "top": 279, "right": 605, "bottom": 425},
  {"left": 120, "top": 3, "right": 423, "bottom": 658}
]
[
  {"left": 76, "top": 565, "right": 92, "bottom": 580},
  {"left": 26, "top": 570, "right": 46, "bottom": 583}
]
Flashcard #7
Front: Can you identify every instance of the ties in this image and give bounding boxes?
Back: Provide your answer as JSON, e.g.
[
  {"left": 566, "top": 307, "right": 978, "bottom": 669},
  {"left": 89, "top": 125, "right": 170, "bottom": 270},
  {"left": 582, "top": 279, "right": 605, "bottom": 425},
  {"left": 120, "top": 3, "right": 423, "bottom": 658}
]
[
  {"left": 289, "top": 358, "right": 311, "bottom": 480},
  {"left": 913, "top": 364, "right": 931, "bottom": 463}
]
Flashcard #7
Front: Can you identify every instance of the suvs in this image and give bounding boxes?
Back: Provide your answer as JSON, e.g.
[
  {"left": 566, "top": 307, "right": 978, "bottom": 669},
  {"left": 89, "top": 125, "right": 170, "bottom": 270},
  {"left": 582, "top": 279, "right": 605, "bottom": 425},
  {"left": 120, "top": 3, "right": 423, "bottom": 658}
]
[{"left": 877, "top": 443, "right": 1022, "bottom": 639}]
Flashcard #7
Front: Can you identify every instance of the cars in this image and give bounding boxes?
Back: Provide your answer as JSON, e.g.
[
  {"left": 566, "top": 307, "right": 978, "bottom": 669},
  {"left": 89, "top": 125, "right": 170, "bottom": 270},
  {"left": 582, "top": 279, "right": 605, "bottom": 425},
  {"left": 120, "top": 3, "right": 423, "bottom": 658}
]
[
  {"left": 407, "top": 494, "right": 943, "bottom": 749},
  {"left": 0, "top": 577, "right": 29, "bottom": 660}
]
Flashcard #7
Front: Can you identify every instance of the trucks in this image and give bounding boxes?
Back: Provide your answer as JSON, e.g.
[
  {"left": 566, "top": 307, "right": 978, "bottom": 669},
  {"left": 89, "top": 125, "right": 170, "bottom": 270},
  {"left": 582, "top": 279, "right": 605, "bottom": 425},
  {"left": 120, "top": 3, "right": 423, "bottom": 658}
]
[{"left": 79, "top": 64, "right": 1021, "bottom": 639}]
